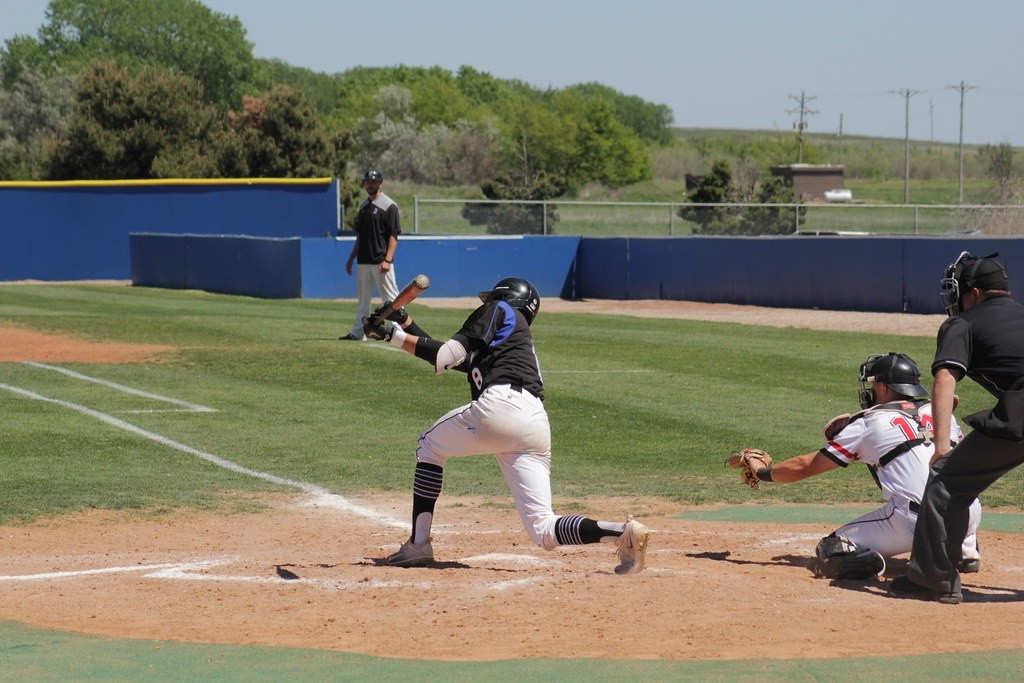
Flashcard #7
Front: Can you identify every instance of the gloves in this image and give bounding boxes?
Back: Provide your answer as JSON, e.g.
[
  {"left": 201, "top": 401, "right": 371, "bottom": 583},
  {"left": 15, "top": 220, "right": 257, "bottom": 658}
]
[
  {"left": 372, "top": 303, "right": 408, "bottom": 325},
  {"left": 361, "top": 315, "right": 407, "bottom": 349}
]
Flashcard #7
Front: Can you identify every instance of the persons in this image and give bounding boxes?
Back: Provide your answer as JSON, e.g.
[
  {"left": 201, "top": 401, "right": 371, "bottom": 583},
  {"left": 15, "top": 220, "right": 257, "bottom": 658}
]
[
  {"left": 338, "top": 169, "right": 405, "bottom": 341},
  {"left": 884, "top": 250, "right": 1024, "bottom": 604},
  {"left": 363, "top": 277, "right": 650, "bottom": 574},
  {"left": 726, "top": 351, "right": 982, "bottom": 582}
]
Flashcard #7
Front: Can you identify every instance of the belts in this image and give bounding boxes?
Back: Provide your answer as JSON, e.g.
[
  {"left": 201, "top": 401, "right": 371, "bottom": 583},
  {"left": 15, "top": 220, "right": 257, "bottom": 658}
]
[{"left": 510, "top": 383, "right": 522, "bottom": 394}]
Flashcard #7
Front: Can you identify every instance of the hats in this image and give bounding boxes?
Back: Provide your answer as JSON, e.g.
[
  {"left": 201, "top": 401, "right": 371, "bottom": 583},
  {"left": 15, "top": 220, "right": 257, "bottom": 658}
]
[{"left": 959, "top": 259, "right": 1009, "bottom": 294}]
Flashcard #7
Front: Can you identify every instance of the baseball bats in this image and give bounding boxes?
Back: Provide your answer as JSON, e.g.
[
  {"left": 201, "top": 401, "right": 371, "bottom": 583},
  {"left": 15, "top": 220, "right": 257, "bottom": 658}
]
[{"left": 375, "top": 274, "right": 430, "bottom": 327}]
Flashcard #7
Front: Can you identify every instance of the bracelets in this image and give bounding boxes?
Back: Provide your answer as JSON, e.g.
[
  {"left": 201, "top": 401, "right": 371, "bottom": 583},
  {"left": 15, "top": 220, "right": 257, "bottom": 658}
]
[{"left": 384, "top": 257, "right": 393, "bottom": 263}]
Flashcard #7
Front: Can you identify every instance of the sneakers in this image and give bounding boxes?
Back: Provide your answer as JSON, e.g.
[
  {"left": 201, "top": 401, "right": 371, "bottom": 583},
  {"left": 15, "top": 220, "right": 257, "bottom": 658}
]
[
  {"left": 613, "top": 514, "right": 651, "bottom": 576},
  {"left": 385, "top": 536, "right": 434, "bottom": 567},
  {"left": 887, "top": 575, "right": 964, "bottom": 605}
]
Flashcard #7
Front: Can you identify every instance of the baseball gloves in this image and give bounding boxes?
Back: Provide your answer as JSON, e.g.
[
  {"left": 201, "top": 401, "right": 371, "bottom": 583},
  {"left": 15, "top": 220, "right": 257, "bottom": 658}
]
[{"left": 728, "top": 447, "right": 773, "bottom": 486}]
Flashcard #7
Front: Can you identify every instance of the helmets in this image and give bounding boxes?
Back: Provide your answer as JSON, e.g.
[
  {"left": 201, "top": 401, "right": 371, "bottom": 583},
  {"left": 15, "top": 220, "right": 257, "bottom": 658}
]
[
  {"left": 364, "top": 171, "right": 382, "bottom": 182},
  {"left": 872, "top": 352, "right": 930, "bottom": 397},
  {"left": 478, "top": 277, "right": 540, "bottom": 326}
]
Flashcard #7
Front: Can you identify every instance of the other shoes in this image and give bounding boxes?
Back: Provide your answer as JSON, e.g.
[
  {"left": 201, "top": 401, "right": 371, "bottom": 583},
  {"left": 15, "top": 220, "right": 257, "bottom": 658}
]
[
  {"left": 338, "top": 333, "right": 358, "bottom": 341},
  {"left": 961, "top": 559, "right": 980, "bottom": 572},
  {"left": 823, "top": 552, "right": 882, "bottom": 579}
]
